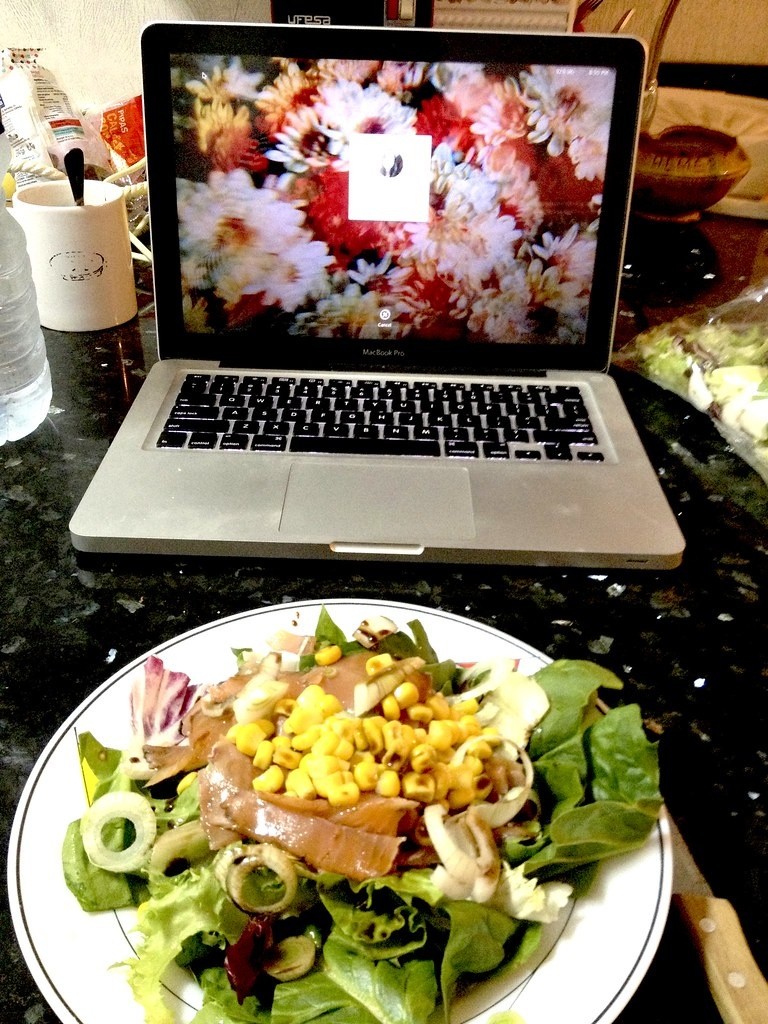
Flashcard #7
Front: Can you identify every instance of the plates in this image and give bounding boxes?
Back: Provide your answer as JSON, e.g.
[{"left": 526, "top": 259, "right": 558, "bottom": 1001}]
[{"left": 3, "top": 594, "right": 674, "bottom": 1023}]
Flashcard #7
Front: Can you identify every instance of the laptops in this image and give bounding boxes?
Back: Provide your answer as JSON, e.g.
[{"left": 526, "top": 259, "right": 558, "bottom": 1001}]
[{"left": 65, "top": 19, "right": 686, "bottom": 575}]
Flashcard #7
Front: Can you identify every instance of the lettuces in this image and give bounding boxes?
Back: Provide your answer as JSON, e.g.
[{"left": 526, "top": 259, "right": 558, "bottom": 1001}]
[{"left": 59, "top": 609, "right": 660, "bottom": 1024}]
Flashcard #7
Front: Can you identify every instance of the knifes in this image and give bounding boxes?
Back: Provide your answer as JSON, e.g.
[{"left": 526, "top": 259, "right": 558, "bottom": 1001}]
[{"left": 665, "top": 822, "right": 768, "bottom": 1021}]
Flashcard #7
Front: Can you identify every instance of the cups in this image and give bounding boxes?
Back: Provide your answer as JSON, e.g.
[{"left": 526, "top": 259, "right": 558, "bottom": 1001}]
[{"left": 13, "top": 179, "right": 139, "bottom": 334}]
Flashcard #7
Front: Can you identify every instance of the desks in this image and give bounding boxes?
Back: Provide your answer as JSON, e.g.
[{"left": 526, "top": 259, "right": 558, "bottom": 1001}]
[{"left": 0, "top": 230, "right": 768, "bottom": 1024}]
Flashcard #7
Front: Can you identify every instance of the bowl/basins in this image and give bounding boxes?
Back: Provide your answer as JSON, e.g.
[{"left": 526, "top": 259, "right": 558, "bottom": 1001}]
[{"left": 631, "top": 124, "right": 751, "bottom": 222}]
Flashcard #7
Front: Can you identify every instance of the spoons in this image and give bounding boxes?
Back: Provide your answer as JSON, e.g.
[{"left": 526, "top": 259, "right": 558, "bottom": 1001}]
[{"left": 64, "top": 147, "right": 86, "bottom": 210}]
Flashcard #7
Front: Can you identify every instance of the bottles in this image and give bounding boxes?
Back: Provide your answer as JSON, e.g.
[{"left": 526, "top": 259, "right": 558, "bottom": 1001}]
[{"left": 0, "top": 180, "right": 54, "bottom": 452}]
